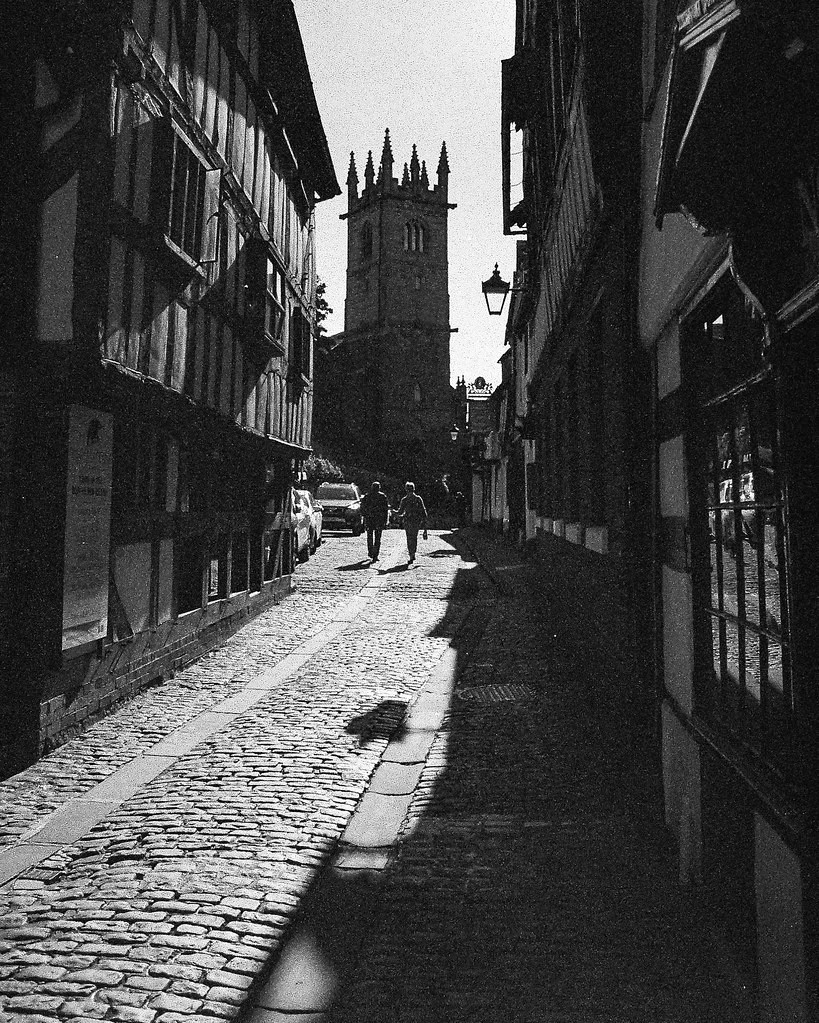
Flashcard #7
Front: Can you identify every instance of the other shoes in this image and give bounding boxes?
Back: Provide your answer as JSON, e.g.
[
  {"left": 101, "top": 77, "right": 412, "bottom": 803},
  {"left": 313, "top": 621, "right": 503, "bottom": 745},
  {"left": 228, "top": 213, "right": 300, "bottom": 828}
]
[{"left": 368, "top": 552, "right": 379, "bottom": 563}]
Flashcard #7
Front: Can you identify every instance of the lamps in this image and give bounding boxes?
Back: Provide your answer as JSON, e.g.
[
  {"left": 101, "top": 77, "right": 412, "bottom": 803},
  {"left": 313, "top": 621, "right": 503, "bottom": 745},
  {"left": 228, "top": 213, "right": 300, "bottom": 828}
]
[
  {"left": 480, "top": 260, "right": 531, "bottom": 316},
  {"left": 450, "top": 424, "right": 484, "bottom": 443}
]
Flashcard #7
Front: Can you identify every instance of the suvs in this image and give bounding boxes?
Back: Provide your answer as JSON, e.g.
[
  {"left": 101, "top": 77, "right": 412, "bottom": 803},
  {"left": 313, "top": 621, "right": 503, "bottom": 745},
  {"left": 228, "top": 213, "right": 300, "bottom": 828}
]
[{"left": 315, "top": 482, "right": 365, "bottom": 536}]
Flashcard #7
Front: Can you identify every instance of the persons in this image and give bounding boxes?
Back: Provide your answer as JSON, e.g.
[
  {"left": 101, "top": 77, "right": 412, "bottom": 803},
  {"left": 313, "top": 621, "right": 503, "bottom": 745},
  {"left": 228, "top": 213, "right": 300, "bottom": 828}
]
[
  {"left": 391, "top": 482, "right": 429, "bottom": 564},
  {"left": 455, "top": 491, "right": 469, "bottom": 529},
  {"left": 360, "top": 481, "right": 391, "bottom": 562}
]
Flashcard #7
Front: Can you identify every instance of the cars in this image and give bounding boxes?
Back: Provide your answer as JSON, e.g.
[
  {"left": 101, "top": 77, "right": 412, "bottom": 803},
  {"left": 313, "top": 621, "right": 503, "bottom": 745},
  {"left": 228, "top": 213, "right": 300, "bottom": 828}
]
[
  {"left": 290, "top": 487, "right": 315, "bottom": 563},
  {"left": 296, "top": 490, "right": 324, "bottom": 550}
]
